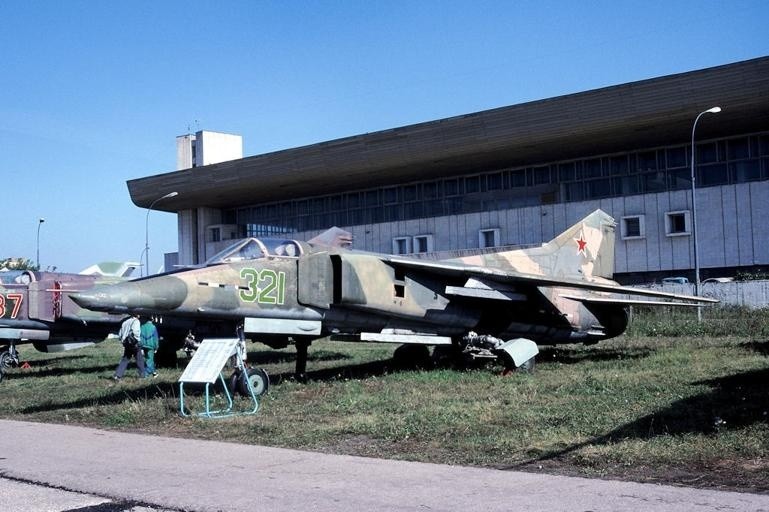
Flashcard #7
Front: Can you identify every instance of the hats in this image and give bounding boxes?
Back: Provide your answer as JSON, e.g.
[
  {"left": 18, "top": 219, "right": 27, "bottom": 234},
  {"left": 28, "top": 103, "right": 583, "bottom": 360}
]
[{"left": 701, "top": 276, "right": 734, "bottom": 283}]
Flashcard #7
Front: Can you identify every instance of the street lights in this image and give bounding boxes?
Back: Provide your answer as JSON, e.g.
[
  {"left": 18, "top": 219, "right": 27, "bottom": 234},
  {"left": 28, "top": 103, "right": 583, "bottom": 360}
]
[{"left": 125, "top": 331, "right": 138, "bottom": 345}]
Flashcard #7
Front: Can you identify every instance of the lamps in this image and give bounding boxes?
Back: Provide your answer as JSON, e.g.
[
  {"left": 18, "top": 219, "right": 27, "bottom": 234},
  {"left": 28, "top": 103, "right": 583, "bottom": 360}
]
[
  {"left": 15, "top": 274, "right": 29, "bottom": 284},
  {"left": 276, "top": 244, "right": 295, "bottom": 256}
]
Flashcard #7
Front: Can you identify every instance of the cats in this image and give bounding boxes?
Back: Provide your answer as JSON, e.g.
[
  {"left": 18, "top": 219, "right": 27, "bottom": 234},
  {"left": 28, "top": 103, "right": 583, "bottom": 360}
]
[
  {"left": 64, "top": 206, "right": 723, "bottom": 395},
  {"left": 0, "top": 222, "right": 356, "bottom": 368}
]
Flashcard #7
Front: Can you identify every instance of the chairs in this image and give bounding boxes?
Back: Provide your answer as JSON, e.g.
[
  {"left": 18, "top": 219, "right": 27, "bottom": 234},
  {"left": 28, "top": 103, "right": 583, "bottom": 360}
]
[
  {"left": 113, "top": 375, "right": 121, "bottom": 381},
  {"left": 153, "top": 373, "right": 159, "bottom": 378}
]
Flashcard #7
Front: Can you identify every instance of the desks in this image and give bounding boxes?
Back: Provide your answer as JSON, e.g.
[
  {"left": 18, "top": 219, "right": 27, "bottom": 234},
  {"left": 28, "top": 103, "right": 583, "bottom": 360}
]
[
  {"left": 36, "top": 217, "right": 48, "bottom": 277},
  {"left": 689, "top": 102, "right": 729, "bottom": 326},
  {"left": 146, "top": 191, "right": 183, "bottom": 277},
  {"left": 139, "top": 246, "right": 151, "bottom": 276}
]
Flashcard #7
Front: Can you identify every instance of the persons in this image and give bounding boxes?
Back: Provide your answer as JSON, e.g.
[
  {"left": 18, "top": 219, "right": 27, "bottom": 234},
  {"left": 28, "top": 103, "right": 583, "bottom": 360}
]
[
  {"left": 140, "top": 315, "right": 161, "bottom": 380},
  {"left": 112, "top": 311, "right": 147, "bottom": 381}
]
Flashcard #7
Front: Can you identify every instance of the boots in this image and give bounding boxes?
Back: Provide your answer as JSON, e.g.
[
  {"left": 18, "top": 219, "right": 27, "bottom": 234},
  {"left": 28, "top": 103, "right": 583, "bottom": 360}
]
[{"left": 661, "top": 275, "right": 690, "bottom": 286}]
